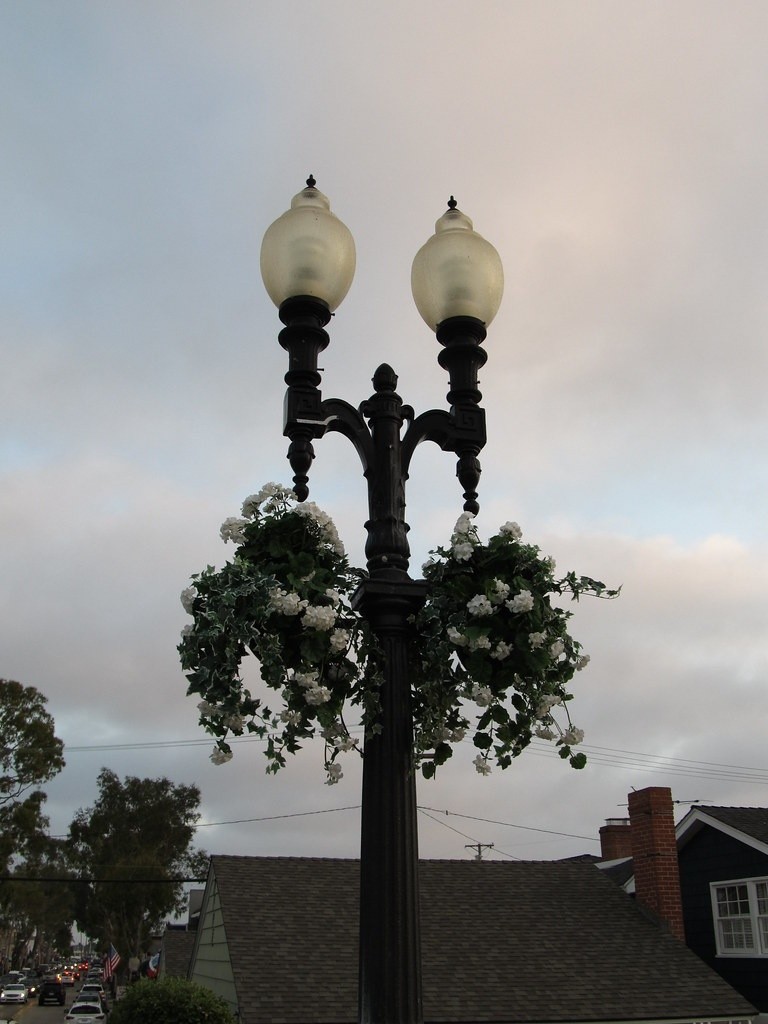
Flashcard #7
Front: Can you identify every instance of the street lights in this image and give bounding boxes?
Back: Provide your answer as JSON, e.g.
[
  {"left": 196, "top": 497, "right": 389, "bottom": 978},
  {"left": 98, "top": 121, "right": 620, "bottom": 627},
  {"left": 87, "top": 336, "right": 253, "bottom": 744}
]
[{"left": 255, "top": 172, "right": 508, "bottom": 1024}]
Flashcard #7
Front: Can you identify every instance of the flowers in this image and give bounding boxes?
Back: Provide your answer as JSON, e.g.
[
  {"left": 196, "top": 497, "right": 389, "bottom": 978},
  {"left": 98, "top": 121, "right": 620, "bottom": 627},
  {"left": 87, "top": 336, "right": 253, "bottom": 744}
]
[
  {"left": 411, "top": 510, "right": 624, "bottom": 775},
  {"left": 171, "top": 484, "right": 372, "bottom": 788}
]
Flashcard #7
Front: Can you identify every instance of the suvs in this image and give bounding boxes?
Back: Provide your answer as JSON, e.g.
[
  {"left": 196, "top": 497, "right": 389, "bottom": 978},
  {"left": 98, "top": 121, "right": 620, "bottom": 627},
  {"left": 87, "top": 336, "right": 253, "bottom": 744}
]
[
  {"left": 76, "top": 982, "right": 107, "bottom": 1001},
  {"left": 39, "top": 982, "right": 66, "bottom": 1006}
]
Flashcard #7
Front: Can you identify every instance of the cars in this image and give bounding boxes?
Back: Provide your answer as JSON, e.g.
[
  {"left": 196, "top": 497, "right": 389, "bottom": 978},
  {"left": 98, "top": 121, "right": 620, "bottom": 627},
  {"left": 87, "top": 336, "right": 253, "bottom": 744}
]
[
  {"left": 64, "top": 1001, "right": 109, "bottom": 1024},
  {"left": 61, "top": 972, "right": 75, "bottom": 987},
  {"left": 0, "top": 984, "right": 29, "bottom": 1004},
  {"left": 0, "top": 968, "right": 43, "bottom": 983},
  {"left": 20, "top": 979, "right": 37, "bottom": 998},
  {"left": 33, "top": 979, "right": 43, "bottom": 993},
  {"left": 74, "top": 991, "right": 105, "bottom": 1009},
  {"left": 39, "top": 956, "right": 107, "bottom": 983}
]
